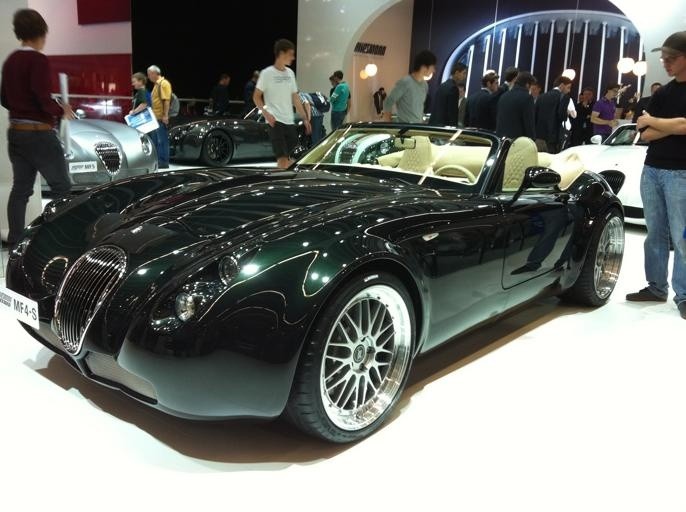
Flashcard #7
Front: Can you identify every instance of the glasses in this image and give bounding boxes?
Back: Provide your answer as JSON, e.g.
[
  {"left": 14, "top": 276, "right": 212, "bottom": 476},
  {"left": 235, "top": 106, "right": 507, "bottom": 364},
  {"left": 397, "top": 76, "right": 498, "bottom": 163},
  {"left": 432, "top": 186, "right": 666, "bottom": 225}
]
[{"left": 659, "top": 56, "right": 682, "bottom": 64}]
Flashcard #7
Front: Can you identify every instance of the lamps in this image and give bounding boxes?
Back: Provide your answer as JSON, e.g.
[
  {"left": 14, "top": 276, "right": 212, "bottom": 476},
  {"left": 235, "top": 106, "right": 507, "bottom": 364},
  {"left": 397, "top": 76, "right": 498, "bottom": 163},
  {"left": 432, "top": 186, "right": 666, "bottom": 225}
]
[
  {"left": 615, "top": 19, "right": 634, "bottom": 74},
  {"left": 422, "top": 0, "right": 438, "bottom": 82},
  {"left": 483, "top": 0, "right": 498, "bottom": 78},
  {"left": 558, "top": 0, "right": 578, "bottom": 80},
  {"left": 365, "top": 22, "right": 377, "bottom": 80},
  {"left": 359, "top": 30, "right": 368, "bottom": 81}
]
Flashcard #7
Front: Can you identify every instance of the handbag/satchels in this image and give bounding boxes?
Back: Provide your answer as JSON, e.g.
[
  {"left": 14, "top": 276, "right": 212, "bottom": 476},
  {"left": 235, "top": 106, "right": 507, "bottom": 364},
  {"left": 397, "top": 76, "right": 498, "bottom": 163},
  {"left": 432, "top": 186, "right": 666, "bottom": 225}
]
[
  {"left": 123, "top": 107, "right": 160, "bottom": 135},
  {"left": 559, "top": 126, "right": 574, "bottom": 150}
]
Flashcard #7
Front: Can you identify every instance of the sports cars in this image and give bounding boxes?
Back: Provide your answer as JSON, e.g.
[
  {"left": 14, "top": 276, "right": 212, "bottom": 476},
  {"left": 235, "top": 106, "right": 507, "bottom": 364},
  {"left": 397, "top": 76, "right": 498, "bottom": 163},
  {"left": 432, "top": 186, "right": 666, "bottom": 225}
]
[
  {"left": 164, "top": 102, "right": 277, "bottom": 168},
  {"left": 38, "top": 95, "right": 157, "bottom": 195},
  {"left": 557, "top": 124, "right": 648, "bottom": 217},
  {"left": 4, "top": 120, "right": 627, "bottom": 446}
]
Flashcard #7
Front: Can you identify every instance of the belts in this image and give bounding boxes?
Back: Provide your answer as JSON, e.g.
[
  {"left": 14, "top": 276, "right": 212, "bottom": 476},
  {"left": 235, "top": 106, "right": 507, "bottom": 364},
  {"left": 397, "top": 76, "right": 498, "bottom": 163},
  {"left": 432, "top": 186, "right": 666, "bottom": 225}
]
[{"left": 9, "top": 123, "right": 51, "bottom": 132}]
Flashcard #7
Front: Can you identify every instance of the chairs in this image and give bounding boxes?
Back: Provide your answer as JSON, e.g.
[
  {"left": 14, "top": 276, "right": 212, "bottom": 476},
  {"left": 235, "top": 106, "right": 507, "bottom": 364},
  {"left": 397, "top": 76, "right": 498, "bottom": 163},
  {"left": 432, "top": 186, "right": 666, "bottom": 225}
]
[
  {"left": 503, "top": 135, "right": 536, "bottom": 190},
  {"left": 394, "top": 132, "right": 434, "bottom": 175}
]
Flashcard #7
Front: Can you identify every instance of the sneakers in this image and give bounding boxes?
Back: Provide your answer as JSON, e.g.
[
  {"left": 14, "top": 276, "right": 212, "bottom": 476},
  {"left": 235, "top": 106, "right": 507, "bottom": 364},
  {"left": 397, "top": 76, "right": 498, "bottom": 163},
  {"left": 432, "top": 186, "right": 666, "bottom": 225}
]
[
  {"left": 678, "top": 302, "right": 686, "bottom": 319},
  {"left": 625, "top": 287, "right": 668, "bottom": 301}
]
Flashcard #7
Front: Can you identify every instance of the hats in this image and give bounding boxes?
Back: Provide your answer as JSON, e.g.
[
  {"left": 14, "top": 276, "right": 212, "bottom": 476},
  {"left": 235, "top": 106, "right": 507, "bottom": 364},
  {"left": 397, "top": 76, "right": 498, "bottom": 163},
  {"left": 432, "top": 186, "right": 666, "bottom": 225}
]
[{"left": 652, "top": 30, "right": 685, "bottom": 54}]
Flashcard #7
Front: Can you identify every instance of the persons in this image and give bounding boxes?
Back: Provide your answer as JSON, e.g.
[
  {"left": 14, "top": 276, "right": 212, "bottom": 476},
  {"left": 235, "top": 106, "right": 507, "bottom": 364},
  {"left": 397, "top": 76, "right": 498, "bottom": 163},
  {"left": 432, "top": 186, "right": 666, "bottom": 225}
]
[
  {"left": 626, "top": 33, "right": 686, "bottom": 321},
  {"left": 252, "top": 39, "right": 311, "bottom": 168},
  {"left": 296, "top": 88, "right": 323, "bottom": 145},
  {"left": 1, "top": 9, "right": 76, "bottom": 251},
  {"left": 329, "top": 75, "right": 352, "bottom": 125},
  {"left": 330, "top": 70, "right": 349, "bottom": 130},
  {"left": 147, "top": 64, "right": 172, "bottom": 168},
  {"left": 213, "top": 73, "right": 230, "bottom": 119},
  {"left": 128, "top": 72, "right": 152, "bottom": 115},
  {"left": 242, "top": 70, "right": 260, "bottom": 118},
  {"left": 374, "top": 52, "right": 662, "bottom": 153}
]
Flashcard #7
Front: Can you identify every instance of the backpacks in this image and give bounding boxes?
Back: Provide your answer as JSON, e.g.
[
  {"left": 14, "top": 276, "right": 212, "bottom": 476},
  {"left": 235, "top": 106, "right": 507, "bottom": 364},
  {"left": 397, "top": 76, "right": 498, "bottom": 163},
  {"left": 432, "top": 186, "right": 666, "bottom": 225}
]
[
  {"left": 310, "top": 91, "right": 331, "bottom": 112},
  {"left": 158, "top": 79, "right": 180, "bottom": 117}
]
